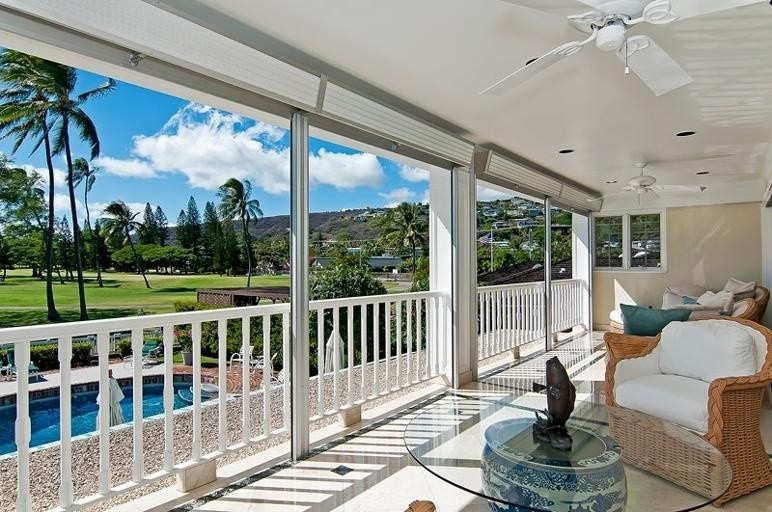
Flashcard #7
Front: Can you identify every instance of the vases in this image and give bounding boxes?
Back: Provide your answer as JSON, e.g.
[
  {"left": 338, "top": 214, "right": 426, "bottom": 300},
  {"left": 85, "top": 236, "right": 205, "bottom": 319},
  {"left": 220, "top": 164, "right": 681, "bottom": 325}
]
[{"left": 181, "top": 349, "right": 193, "bottom": 366}]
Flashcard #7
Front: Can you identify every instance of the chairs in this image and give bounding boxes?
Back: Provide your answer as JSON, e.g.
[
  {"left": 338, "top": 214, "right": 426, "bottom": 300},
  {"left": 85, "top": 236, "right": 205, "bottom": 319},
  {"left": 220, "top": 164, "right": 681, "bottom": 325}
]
[
  {"left": 4, "top": 345, "right": 42, "bottom": 386},
  {"left": 229, "top": 343, "right": 286, "bottom": 387},
  {"left": 600, "top": 276, "right": 771, "bottom": 509},
  {"left": 133, "top": 338, "right": 161, "bottom": 366}
]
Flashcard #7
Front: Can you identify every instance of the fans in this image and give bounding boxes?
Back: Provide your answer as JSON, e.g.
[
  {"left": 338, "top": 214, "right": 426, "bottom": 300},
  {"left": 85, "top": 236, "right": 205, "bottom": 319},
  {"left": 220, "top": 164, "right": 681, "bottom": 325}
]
[
  {"left": 475, "top": 0, "right": 771, "bottom": 105},
  {"left": 582, "top": 159, "right": 710, "bottom": 210}
]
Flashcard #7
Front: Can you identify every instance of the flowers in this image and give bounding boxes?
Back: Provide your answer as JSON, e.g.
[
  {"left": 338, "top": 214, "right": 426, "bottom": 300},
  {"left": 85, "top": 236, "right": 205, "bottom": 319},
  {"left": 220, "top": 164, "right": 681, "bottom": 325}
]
[{"left": 173, "top": 328, "right": 194, "bottom": 348}]
